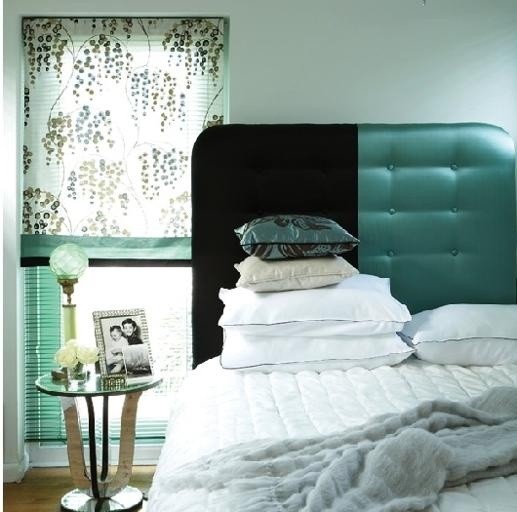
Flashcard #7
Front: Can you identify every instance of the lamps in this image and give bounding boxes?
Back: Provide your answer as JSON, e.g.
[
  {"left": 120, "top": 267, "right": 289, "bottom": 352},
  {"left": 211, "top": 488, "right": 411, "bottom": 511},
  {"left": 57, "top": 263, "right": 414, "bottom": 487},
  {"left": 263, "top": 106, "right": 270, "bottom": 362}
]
[{"left": 49, "top": 242, "right": 88, "bottom": 372}]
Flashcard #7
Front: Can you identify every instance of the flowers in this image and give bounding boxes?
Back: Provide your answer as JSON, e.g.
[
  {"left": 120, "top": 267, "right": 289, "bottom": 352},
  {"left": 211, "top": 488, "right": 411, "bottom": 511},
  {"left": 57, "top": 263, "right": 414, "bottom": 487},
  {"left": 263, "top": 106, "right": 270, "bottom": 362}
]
[{"left": 55, "top": 339, "right": 99, "bottom": 367}]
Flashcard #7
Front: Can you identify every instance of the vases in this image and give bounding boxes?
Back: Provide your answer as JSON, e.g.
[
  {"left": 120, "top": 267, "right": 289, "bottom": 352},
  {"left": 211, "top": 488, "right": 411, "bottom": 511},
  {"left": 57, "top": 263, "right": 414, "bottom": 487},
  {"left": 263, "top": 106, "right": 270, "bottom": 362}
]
[{"left": 67, "top": 362, "right": 87, "bottom": 387}]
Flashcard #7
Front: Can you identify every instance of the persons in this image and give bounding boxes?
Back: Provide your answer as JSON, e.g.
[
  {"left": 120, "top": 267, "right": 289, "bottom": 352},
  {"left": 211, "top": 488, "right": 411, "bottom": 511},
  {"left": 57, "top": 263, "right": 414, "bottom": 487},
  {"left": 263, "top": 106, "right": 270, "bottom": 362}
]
[
  {"left": 121, "top": 318, "right": 143, "bottom": 345},
  {"left": 106, "top": 325, "right": 129, "bottom": 373}
]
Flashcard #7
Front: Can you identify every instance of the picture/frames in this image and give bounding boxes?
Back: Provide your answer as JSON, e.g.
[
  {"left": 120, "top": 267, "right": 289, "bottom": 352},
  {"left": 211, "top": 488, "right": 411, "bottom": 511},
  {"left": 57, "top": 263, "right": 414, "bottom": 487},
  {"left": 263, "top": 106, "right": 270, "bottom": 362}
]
[{"left": 93, "top": 308, "right": 154, "bottom": 378}]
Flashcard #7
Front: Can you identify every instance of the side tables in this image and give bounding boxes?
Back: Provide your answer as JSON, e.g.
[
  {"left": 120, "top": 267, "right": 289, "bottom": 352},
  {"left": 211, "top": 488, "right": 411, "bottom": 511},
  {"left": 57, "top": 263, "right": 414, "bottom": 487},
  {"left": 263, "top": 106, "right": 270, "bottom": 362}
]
[{"left": 35, "top": 364, "right": 165, "bottom": 512}]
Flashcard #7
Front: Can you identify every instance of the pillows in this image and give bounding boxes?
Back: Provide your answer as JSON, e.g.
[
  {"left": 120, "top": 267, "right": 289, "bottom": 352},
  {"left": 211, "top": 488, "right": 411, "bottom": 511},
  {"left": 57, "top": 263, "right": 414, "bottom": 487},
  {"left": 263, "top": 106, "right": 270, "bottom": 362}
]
[
  {"left": 219, "top": 273, "right": 412, "bottom": 337},
  {"left": 402, "top": 304, "right": 516, "bottom": 365},
  {"left": 234, "top": 255, "right": 358, "bottom": 292},
  {"left": 220, "top": 329, "right": 414, "bottom": 373},
  {"left": 235, "top": 214, "right": 361, "bottom": 260}
]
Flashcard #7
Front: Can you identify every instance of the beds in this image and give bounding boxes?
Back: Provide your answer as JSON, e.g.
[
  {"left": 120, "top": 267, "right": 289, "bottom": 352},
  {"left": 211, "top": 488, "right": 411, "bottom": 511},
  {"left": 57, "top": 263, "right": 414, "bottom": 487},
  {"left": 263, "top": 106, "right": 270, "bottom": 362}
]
[{"left": 148, "top": 124, "right": 516, "bottom": 512}]
